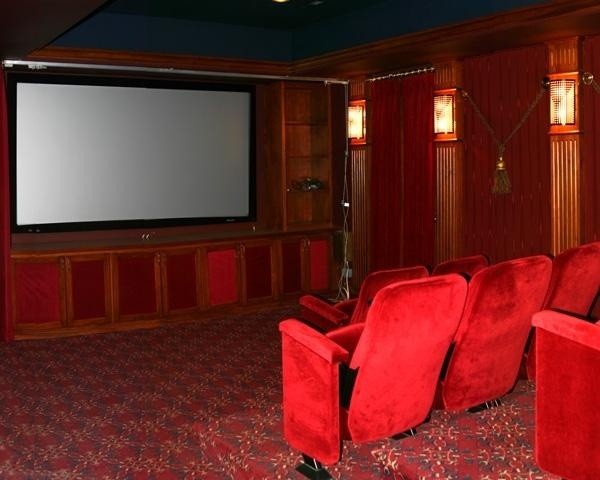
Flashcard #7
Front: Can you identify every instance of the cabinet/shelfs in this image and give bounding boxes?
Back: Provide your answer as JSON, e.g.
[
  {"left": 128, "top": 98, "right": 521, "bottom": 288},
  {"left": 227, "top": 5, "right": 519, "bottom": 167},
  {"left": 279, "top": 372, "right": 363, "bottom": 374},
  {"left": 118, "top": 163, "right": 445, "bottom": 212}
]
[{"left": 270, "top": 80, "right": 334, "bottom": 234}]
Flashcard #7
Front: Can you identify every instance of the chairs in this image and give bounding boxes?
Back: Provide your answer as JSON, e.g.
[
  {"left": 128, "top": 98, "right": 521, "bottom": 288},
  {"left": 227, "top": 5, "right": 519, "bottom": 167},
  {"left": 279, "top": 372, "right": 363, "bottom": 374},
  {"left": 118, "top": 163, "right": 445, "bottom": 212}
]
[{"left": 277, "top": 240, "right": 599, "bottom": 478}]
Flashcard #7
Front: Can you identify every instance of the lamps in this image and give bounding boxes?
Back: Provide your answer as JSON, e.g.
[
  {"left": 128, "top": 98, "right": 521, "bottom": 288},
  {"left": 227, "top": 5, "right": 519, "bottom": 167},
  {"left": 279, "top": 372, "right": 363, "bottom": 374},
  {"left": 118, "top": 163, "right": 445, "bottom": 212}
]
[{"left": 341, "top": 71, "right": 582, "bottom": 145}]
